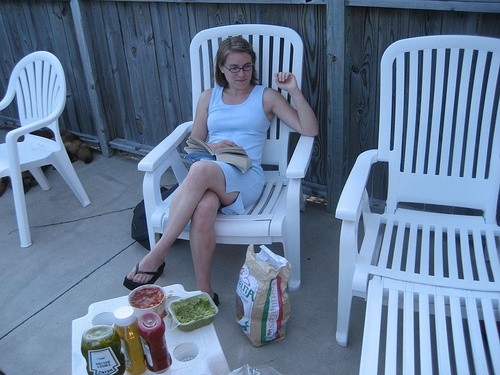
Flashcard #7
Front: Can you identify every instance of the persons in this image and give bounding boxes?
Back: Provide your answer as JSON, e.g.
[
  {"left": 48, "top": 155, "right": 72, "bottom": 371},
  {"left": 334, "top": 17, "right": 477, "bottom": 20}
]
[{"left": 122, "top": 36, "right": 318, "bottom": 304}]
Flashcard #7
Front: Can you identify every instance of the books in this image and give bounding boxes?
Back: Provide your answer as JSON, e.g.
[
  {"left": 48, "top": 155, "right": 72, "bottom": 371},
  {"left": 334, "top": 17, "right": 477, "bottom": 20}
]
[{"left": 184, "top": 134, "right": 253, "bottom": 174}]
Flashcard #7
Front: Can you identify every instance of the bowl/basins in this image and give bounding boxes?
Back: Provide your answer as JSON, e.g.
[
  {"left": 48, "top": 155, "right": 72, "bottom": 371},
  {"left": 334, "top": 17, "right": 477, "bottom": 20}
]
[
  {"left": 168, "top": 292, "right": 219, "bottom": 332},
  {"left": 128, "top": 284, "right": 167, "bottom": 316}
]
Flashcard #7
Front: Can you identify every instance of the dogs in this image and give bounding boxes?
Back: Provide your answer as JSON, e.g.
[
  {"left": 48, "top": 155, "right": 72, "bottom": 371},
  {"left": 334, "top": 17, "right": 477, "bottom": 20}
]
[{"left": 0, "top": 127, "right": 97, "bottom": 196}]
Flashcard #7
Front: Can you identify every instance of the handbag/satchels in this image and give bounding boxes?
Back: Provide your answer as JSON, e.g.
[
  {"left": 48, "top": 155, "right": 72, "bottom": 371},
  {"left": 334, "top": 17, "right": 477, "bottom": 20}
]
[{"left": 132, "top": 183, "right": 179, "bottom": 250}]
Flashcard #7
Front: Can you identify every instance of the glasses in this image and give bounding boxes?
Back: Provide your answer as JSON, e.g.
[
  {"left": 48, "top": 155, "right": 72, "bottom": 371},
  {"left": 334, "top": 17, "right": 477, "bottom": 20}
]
[{"left": 222, "top": 64, "right": 252, "bottom": 73}]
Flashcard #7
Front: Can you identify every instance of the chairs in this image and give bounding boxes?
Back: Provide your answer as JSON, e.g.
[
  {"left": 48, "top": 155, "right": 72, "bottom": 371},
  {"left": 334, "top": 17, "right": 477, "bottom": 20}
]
[
  {"left": 334, "top": 34, "right": 500, "bottom": 375},
  {"left": 0, "top": 51, "right": 91, "bottom": 248},
  {"left": 138, "top": 23, "right": 315, "bottom": 291}
]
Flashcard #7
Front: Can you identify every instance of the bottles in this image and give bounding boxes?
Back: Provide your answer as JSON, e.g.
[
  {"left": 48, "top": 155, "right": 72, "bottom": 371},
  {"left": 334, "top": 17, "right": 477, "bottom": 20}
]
[
  {"left": 112, "top": 306, "right": 147, "bottom": 375},
  {"left": 81, "top": 325, "right": 126, "bottom": 375},
  {"left": 137, "top": 312, "right": 171, "bottom": 375}
]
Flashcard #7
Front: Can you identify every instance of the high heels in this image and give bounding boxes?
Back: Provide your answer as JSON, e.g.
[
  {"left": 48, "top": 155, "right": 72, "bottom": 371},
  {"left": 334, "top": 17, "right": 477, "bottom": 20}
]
[
  {"left": 123, "top": 259, "right": 165, "bottom": 290},
  {"left": 212, "top": 293, "right": 219, "bottom": 306}
]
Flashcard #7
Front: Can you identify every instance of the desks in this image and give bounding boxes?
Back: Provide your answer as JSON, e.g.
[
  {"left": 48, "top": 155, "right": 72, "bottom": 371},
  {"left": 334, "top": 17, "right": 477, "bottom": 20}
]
[{"left": 71, "top": 283, "right": 230, "bottom": 375}]
